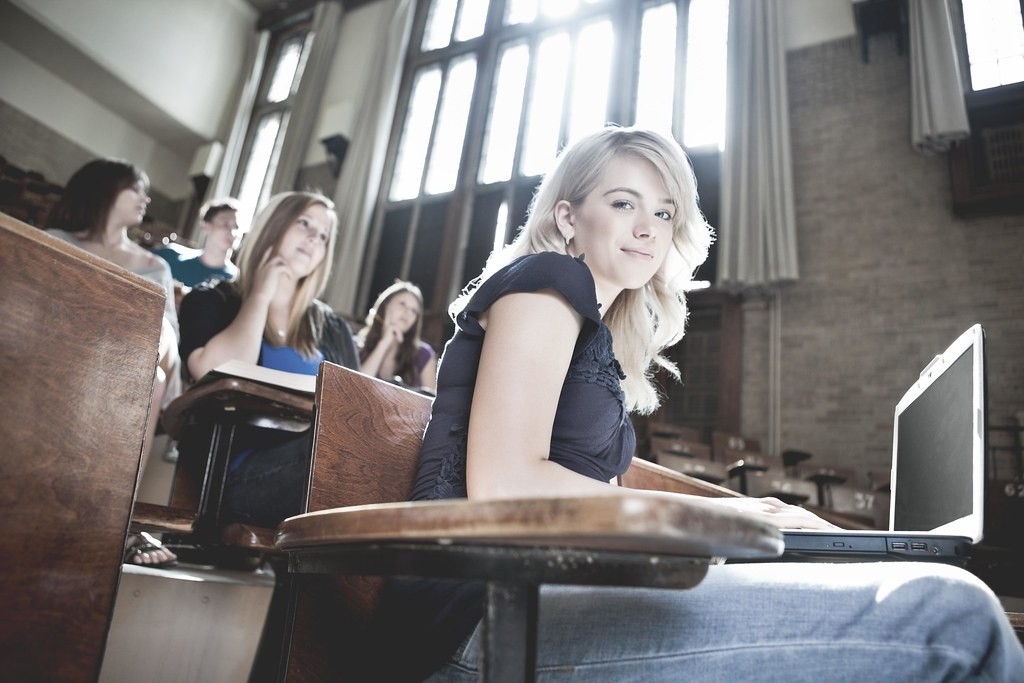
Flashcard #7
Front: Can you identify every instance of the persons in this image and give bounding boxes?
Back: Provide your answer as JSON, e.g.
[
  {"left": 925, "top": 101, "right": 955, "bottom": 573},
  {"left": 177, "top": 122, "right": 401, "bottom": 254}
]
[
  {"left": 382, "top": 123, "right": 1023, "bottom": 683},
  {"left": 351, "top": 281, "right": 438, "bottom": 398},
  {"left": 150, "top": 193, "right": 246, "bottom": 292},
  {"left": 176, "top": 187, "right": 367, "bottom": 572},
  {"left": 37, "top": 155, "right": 181, "bottom": 565}
]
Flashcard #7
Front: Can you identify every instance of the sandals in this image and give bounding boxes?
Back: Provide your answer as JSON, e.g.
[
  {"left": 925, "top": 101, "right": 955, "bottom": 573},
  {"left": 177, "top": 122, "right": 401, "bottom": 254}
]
[{"left": 123, "top": 531, "right": 178, "bottom": 568}]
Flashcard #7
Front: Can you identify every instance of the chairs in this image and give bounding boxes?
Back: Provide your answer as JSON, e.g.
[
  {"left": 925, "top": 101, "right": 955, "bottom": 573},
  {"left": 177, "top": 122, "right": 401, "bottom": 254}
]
[{"left": 1, "top": 208, "right": 892, "bottom": 683}]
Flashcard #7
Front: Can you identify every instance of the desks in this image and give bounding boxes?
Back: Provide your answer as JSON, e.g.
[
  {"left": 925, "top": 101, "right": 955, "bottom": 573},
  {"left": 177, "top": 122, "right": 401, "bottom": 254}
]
[
  {"left": 273, "top": 490, "right": 784, "bottom": 683},
  {"left": 159, "top": 378, "right": 316, "bottom": 564}
]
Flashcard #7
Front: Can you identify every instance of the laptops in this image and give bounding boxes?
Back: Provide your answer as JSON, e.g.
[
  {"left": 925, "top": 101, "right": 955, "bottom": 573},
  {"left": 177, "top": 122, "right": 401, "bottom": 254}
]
[{"left": 777, "top": 324, "right": 985, "bottom": 553}]
